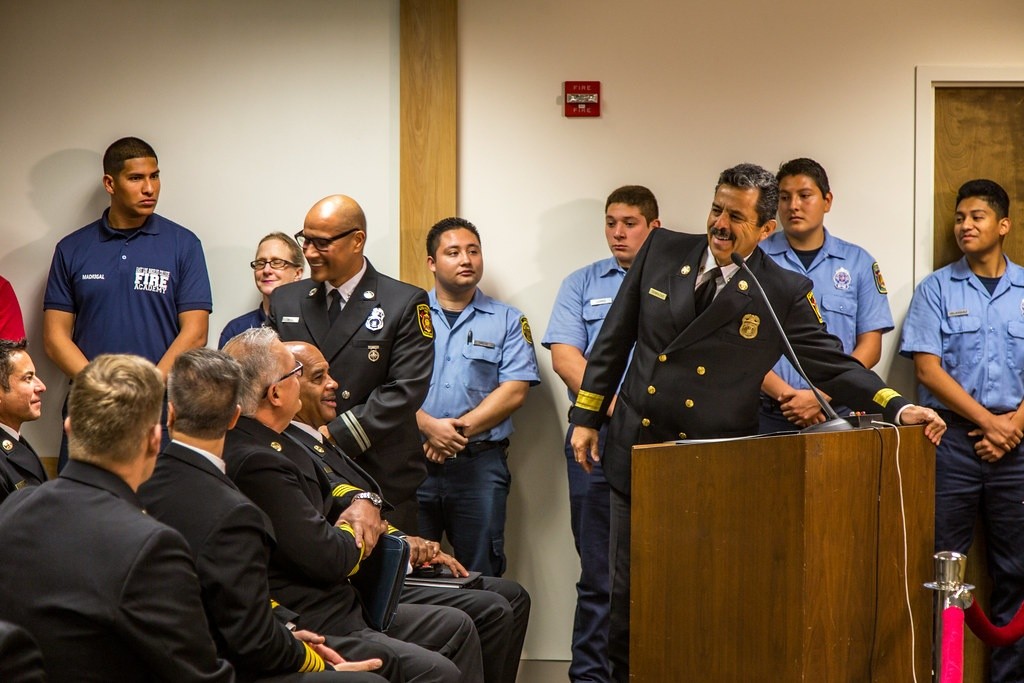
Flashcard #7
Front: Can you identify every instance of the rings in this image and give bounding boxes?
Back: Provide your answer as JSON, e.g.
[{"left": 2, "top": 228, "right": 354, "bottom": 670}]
[{"left": 425, "top": 541, "right": 431, "bottom": 545}]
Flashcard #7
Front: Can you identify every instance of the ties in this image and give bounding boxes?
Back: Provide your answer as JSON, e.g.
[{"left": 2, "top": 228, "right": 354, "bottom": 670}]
[
  {"left": 19, "top": 435, "right": 27, "bottom": 448},
  {"left": 321, "top": 434, "right": 340, "bottom": 457},
  {"left": 694, "top": 267, "right": 722, "bottom": 317},
  {"left": 328, "top": 288, "right": 342, "bottom": 327}
]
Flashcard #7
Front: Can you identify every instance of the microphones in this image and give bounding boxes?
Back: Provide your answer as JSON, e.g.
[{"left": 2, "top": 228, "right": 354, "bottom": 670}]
[{"left": 730, "top": 251, "right": 854, "bottom": 434}]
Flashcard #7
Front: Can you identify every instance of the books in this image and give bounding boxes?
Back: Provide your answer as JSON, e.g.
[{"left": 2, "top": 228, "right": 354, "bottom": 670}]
[{"left": 405, "top": 568, "right": 482, "bottom": 589}]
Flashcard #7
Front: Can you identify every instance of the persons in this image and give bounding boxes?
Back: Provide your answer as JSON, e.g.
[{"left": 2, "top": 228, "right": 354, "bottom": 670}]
[
  {"left": 566, "top": 165, "right": 945, "bottom": 683},
  {"left": 0, "top": 275, "right": 26, "bottom": 342},
  {"left": 415, "top": 217, "right": 541, "bottom": 578},
  {"left": 756, "top": 158, "right": 895, "bottom": 436},
  {"left": 0, "top": 354, "right": 235, "bottom": 683},
  {"left": 262, "top": 193, "right": 435, "bottom": 533},
  {"left": 43, "top": 138, "right": 212, "bottom": 478},
  {"left": 899, "top": 180, "right": 1023, "bottom": 683},
  {"left": 218, "top": 232, "right": 305, "bottom": 350},
  {"left": 542, "top": 184, "right": 662, "bottom": 558},
  {"left": 219, "top": 324, "right": 484, "bottom": 683},
  {"left": 282, "top": 341, "right": 532, "bottom": 683},
  {"left": 134, "top": 345, "right": 389, "bottom": 683},
  {"left": 0, "top": 338, "right": 48, "bottom": 507}
]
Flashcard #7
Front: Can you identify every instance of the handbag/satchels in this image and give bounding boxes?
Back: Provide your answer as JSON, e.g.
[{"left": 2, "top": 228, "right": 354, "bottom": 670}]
[{"left": 348, "top": 533, "right": 410, "bottom": 632}]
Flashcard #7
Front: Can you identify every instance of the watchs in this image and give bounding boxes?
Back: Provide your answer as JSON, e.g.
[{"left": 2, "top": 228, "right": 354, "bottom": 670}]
[{"left": 352, "top": 491, "right": 383, "bottom": 510}]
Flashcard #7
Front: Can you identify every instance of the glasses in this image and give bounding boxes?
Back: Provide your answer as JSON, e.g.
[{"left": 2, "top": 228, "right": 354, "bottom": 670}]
[
  {"left": 251, "top": 259, "right": 299, "bottom": 270},
  {"left": 262, "top": 360, "right": 305, "bottom": 400},
  {"left": 293, "top": 229, "right": 359, "bottom": 252}
]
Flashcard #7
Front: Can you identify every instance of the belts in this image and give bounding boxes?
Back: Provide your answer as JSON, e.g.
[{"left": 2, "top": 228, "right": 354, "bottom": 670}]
[
  {"left": 471, "top": 440, "right": 501, "bottom": 452},
  {"left": 760, "top": 396, "right": 779, "bottom": 407}
]
